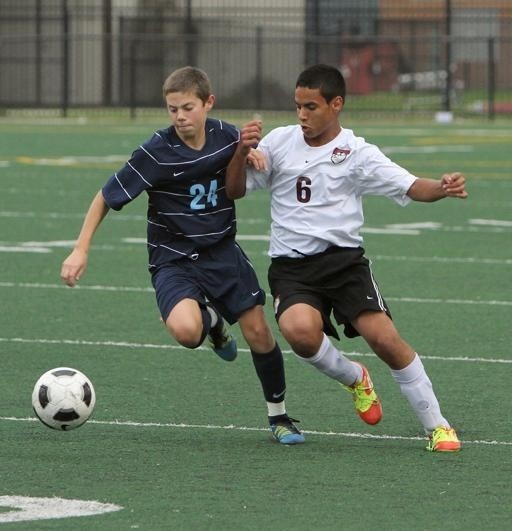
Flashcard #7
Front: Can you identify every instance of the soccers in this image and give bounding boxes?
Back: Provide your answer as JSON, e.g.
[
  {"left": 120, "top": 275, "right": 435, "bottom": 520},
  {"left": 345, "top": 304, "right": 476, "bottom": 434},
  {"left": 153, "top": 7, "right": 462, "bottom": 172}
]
[{"left": 31, "top": 367, "right": 95, "bottom": 431}]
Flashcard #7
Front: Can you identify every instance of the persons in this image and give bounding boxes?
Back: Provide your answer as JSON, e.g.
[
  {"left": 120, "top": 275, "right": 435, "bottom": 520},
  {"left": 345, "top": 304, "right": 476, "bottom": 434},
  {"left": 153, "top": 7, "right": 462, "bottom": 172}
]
[
  {"left": 58, "top": 67, "right": 309, "bottom": 447},
  {"left": 225, "top": 65, "right": 471, "bottom": 455}
]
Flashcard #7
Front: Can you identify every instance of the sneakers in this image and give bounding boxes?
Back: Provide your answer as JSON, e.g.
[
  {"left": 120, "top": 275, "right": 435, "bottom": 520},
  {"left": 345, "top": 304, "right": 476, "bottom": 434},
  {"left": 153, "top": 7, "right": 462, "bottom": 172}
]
[
  {"left": 268, "top": 414, "right": 304, "bottom": 443},
  {"left": 349, "top": 361, "right": 382, "bottom": 425},
  {"left": 426, "top": 427, "right": 462, "bottom": 451},
  {"left": 205, "top": 302, "right": 237, "bottom": 362}
]
